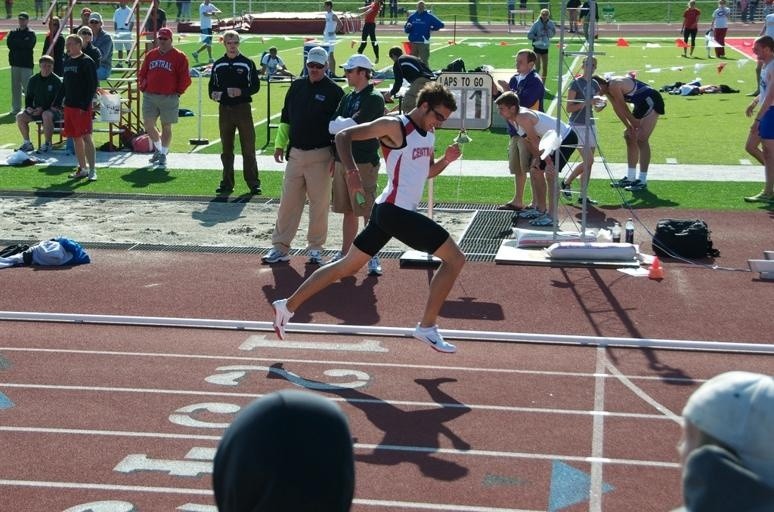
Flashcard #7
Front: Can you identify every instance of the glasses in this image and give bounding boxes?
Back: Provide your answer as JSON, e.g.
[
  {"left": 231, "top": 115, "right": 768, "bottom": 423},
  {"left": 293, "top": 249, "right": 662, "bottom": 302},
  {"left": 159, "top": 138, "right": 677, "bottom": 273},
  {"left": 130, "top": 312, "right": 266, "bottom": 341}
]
[
  {"left": 307, "top": 63, "right": 324, "bottom": 69},
  {"left": 82, "top": 31, "right": 92, "bottom": 36},
  {"left": 90, "top": 21, "right": 99, "bottom": 24},
  {"left": 344, "top": 69, "right": 353, "bottom": 73},
  {"left": 227, "top": 41, "right": 239, "bottom": 45},
  {"left": 432, "top": 109, "right": 448, "bottom": 123}
]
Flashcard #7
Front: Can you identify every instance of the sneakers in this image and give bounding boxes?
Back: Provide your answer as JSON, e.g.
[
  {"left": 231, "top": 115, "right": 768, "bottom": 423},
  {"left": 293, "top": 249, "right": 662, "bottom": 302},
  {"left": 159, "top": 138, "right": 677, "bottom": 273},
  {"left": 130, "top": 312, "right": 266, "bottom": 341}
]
[
  {"left": 412, "top": 321, "right": 457, "bottom": 353},
  {"left": 36, "top": 143, "right": 52, "bottom": 156},
  {"left": 577, "top": 197, "right": 599, "bottom": 206},
  {"left": 209, "top": 58, "right": 215, "bottom": 63},
  {"left": 624, "top": 180, "right": 647, "bottom": 191},
  {"left": 261, "top": 248, "right": 290, "bottom": 263},
  {"left": 560, "top": 181, "right": 573, "bottom": 201},
  {"left": 148, "top": 149, "right": 159, "bottom": 161},
  {"left": 367, "top": 255, "right": 383, "bottom": 276},
  {"left": 746, "top": 89, "right": 759, "bottom": 96},
  {"left": 88, "top": 169, "right": 97, "bottom": 181},
  {"left": 317, "top": 251, "right": 344, "bottom": 267},
  {"left": 528, "top": 213, "right": 560, "bottom": 227},
  {"left": 744, "top": 189, "right": 764, "bottom": 202},
  {"left": 68, "top": 167, "right": 88, "bottom": 178},
  {"left": 14, "top": 143, "right": 34, "bottom": 152},
  {"left": 158, "top": 153, "right": 166, "bottom": 167},
  {"left": 215, "top": 185, "right": 235, "bottom": 194},
  {"left": 251, "top": 186, "right": 262, "bottom": 194},
  {"left": 519, "top": 207, "right": 547, "bottom": 219},
  {"left": 610, "top": 176, "right": 633, "bottom": 188},
  {"left": 191, "top": 51, "right": 198, "bottom": 62},
  {"left": 758, "top": 192, "right": 774, "bottom": 202},
  {"left": 114, "top": 63, "right": 123, "bottom": 67},
  {"left": 271, "top": 298, "right": 294, "bottom": 341},
  {"left": 307, "top": 249, "right": 321, "bottom": 263}
]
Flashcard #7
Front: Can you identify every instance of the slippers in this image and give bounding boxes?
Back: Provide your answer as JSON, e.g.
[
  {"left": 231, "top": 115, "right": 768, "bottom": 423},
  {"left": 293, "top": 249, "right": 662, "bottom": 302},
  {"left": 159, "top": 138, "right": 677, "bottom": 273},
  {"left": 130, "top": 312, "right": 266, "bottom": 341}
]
[
  {"left": 520, "top": 205, "right": 535, "bottom": 211},
  {"left": 497, "top": 201, "right": 523, "bottom": 211}
]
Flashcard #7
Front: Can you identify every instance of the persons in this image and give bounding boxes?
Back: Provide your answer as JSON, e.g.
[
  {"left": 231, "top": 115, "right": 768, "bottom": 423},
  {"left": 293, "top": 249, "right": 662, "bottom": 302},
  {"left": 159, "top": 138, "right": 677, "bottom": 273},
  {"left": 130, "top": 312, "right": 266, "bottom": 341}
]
[
  {"left": 579, "top": 2, "right": 599, "bottom": 44},
  {"left": 208, "top": 30, "right": 262, "bottom": 194},
  {"left": 709, "top": 0, "right": 731, "bottom": 59},
  {"left": 260, "top": 48, "right": 295, "bottom": 78},
  {"left": 559, "top": 57, "right": 606, "bottom": 205},
  {"left": 680, "top": 0, "right": 701, "bottom": 57},
  {"left": 379, "top": 0, "right": 386, "bottom": 26},
  {"left": 144, "top": 0, "right": 166, "bottom": 32},
  {"left": 323, "top": 1, "right": 344, "bottom": 77},
  {"left": 64, "top": 26, "right": 103, "bottom": 156},
  {"left": 493, "top": 91, "right": 579, "bottom": 227},
  {"left": 72, "top": 7, "right": 92, "bottom": 33},
  {"left": 191, "top": 0, "right": 222, "bottom": 64},
  {"left": 668, "top": 371, "right": 774, "bottom": 511},
  {"left": 592, "top": 74, "right": 665, "bottom": 192},
  {"left": 181, "top": 0, "right": 191, "bottom": 22},
  {"left": 385, "top": 47, "right": 438, "bottom": 113},
  {"left": 486, "top": 48, "right": 544, "bottom": 212},
  {"left": 138, "top": 28, "right": 192, "bottom": 165},
  {"left": 113, "top": 0, "right": 134, "bottom": 70},
  {"left": 528, "top": 8, "right": 556, "bottom": 93},
  {"left": 356, "top": 0, "right": 383, "bottom": 64},
  {"left": 328, "top": 53, "right": 385, "bottom": 277},
  {"left": 389, "top": 1, "right": 398, "bottom": 26},
  {"left": 404, "top": 2, "right": 445, "bottom": 66},
  {"left": 6, "top": 12, "right": 38, "bottom": 116},
  {"left": 87, "top": 12, "right": 113, "bottom": 80},
  {"left": 271, "top": 84, "right": 466, "bottom": 353},
  {"left": 744, "top": 36, "right": 774, "bottom": 203},
  {"left": 13, "top": 54, "right": 63, "bottom": 155},
  {"left": 519, "top": 0, "right": 527, "bottom": 26},
  {"left": 261, "top": 46, "right": 346, "bottom": 264},
  {"left": 507, "top": 0, "right": 516, "bottom": 25},
  {"left": 4, "top": 0, "right": 13, "bottom": 19},
  {"left": 176, "top": 0, "right": 182, "bottom": 22},
  {"left": 746, "top": 7, "right": 774, "bottom": 97},
  {"left": 34, "top": 0, "right": 45, "bottom": 20},
  {"left": 566, "top": 0, "right": 580, "bottom": 32},
  {"left": 41, "top": 16, "right": 65, "bottom": 78}
]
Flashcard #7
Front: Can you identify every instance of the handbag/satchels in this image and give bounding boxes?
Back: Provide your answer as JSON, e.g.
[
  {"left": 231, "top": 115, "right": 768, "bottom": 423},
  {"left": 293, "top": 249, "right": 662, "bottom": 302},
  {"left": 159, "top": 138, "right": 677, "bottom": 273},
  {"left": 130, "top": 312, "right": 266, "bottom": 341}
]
[{"left": 652, "top": 218, "right": 720, "bottom": 265}]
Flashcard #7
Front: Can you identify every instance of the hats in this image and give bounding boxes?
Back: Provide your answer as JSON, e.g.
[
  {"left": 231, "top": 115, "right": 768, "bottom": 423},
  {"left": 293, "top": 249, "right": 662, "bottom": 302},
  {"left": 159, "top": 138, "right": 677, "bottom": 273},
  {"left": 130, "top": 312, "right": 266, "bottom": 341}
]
[
  {"left": 307, "top": 47, "right": 328, "bottom": 65},
  {"left": 157, "top": 28, "right": 173, "bottom": 39},
  {"left": 338, "top": 54, "right": 373, "bottom": 69},
  {"left": 89, "top": 12, "right": 102, "bottom": 22},
  {"left": 681, "top": 369, "right": 774, "bottom": 480},
  {"left": 18, "top": 12, "right": 29, "bottom": 20},
  {"left": 81, "top": 8, "right": 91, "bottom": 15}
]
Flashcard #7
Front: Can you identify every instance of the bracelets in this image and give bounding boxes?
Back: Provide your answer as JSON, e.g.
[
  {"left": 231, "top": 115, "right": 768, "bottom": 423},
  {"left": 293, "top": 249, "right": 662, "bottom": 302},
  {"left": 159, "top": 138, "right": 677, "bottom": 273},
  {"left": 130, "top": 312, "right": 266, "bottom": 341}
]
[
  {"left": 755, "top": 118, "right": 761, "bottom": 122},
  {"left": 342, "top": 169, "right": 361, "bottom": 178}
]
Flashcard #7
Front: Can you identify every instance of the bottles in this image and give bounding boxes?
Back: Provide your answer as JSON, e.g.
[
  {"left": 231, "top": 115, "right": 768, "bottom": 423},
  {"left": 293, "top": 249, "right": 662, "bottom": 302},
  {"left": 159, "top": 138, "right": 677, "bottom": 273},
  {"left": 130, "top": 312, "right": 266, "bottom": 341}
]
[
  {"left": 612, "top": 222, "right": 621, "bottom": 243},
  {"left": 625, "top": 217, "right": 635, "bottom": 245}
]
